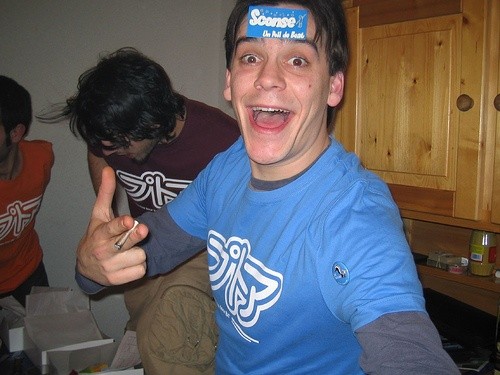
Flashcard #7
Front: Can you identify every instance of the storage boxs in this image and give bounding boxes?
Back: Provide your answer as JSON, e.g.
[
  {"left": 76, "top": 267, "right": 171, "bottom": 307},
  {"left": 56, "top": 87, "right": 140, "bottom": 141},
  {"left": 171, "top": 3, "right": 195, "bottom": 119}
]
[{"left": 427, "top": 251, "right": 458, "bottom": 271}]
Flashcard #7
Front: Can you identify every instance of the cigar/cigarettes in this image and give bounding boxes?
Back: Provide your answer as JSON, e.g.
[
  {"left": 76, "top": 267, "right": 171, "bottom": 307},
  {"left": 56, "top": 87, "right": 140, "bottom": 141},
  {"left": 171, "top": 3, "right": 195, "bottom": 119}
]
[{"left": 114, "top": 219, "right": 139, "bottom": 249}]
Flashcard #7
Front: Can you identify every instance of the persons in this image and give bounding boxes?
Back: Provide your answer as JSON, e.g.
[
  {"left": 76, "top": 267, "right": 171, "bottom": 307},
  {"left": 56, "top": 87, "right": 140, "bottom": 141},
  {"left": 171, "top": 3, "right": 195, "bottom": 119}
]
[
  {"left": 35, "top": 47, "right": 241, "bottom": 375},
  {"left": 76, "top": 0, "right": 462, "bottom": 375},
  {"left": 0, "top": 74, "right": 56, "bottom": 375}
]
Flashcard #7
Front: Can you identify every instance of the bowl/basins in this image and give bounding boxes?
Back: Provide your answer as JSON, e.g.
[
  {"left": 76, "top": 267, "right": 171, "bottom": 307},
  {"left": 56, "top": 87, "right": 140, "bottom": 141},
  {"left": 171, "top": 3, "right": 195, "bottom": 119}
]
[
  {"left": 426, "top": 250, "right": 463, "bottom": 271},
  {"left": 447, "top": 263, "right": 468, "bottom": 275}
]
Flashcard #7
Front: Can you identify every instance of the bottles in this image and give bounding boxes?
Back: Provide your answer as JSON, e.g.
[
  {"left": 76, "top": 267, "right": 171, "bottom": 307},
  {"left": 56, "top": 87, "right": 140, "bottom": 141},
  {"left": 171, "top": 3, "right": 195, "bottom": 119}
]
[{"left": 470, "top": 230, "right": 497, "bottom": 276}]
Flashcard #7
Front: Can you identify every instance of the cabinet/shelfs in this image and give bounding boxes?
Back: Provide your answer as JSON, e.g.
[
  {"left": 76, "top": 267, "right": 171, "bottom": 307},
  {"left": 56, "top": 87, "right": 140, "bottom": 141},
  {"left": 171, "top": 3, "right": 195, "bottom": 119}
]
[{"left": 322, "top": 0, "right": 500, "bottom": 233}]
